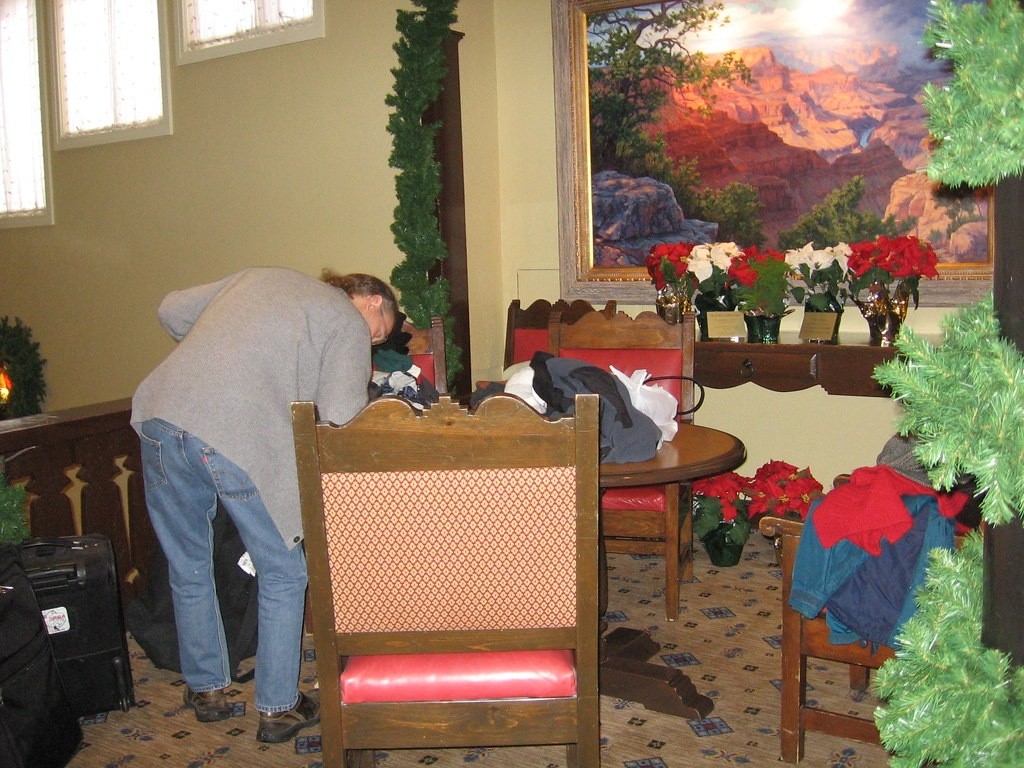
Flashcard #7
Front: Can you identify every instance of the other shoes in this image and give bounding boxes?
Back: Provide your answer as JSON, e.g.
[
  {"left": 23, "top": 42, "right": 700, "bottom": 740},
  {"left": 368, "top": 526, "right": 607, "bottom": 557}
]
[
  {"left": 186, "top": 683, "right": 229, "bottom": 720},
  {"left": 256, "top": 688, "right": 320, "bottom": 742}
]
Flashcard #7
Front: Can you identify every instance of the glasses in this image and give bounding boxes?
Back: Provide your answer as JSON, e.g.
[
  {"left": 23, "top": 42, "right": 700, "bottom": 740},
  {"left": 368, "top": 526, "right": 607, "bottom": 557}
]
[{"left": 371, "top": 305, "right": 388, "bottom": 345}]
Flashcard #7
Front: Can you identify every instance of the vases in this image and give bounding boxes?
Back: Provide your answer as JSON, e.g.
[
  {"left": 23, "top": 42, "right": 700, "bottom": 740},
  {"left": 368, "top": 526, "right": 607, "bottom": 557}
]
[
  {"left": 743, "top": 309, "right": 795, "bottom": 344},
  {"left": 696, "top": 313, "right": 731, "bottom": 341},
  {"left": 804, "top": 299, "right": 844, "bottom": 346},
  {"left": 654, "top": 296, "right": 691, "bottom": 324},
  {"left": 849, "top": 284, "right": 911, "bottom": 344}
]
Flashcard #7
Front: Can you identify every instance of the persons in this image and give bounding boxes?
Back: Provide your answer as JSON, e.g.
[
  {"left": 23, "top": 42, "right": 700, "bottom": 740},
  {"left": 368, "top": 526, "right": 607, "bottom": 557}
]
[{"left": 129, "top": 266, "right": 399, "bottom": 742}]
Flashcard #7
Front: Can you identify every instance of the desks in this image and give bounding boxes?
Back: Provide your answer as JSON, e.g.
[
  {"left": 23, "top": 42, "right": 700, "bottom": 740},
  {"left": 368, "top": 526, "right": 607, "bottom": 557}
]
[
  {"left": 455, "top": 395, "right": 746, "bottom": 720},
  {"left": 693, "top": 331, "right": 944, "bottom": 398}
]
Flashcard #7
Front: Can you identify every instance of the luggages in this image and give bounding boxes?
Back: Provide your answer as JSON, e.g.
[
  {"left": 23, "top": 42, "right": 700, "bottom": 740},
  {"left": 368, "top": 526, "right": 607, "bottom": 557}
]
[
  {"left": 0, "top": 544, "right": 88, "bottom": 768},
  {"left": 15, "top": 535, "right": 133, "bottom": 719}
]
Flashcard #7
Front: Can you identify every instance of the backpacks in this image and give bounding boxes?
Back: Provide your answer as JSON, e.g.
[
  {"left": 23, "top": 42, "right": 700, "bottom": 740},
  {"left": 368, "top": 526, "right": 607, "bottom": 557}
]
[{"left": 119, "top": 495, "right": 259, "bottom": 684}]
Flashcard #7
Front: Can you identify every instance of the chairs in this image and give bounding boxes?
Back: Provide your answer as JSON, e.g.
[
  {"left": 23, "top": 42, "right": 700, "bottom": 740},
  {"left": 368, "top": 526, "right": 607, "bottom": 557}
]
[
  {"left": 548, "top": 311, "right": 695, "bottom": 621},
  {"left": 503, "top": 299, "right": 617, "bottom": 373},
  {"left": 386, "top": 312, "right": 448, "bottom": 393},
  {"left": 758, "top": 472, "right": 967, "bottom": 764},
  {"left": 290, "top": 392, "right": 601, "bottom": 768}
]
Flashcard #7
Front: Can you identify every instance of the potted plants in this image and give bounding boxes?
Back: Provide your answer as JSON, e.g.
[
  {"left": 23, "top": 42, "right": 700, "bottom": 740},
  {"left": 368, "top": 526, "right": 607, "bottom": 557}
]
[{"left": 701, "top": 523, "right": 743, "bottom": 567}]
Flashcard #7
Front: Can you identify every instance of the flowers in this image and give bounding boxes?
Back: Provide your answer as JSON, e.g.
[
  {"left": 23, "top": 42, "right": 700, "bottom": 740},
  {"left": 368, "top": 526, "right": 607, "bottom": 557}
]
[
  {"left": 845, "top": 235, "right": 941, "bottom": 287},
  {"left": 749, "top": 459, "right": 823, "bottom": 524},
  {"left": 724, "top": 245, "right": 796, "bottom": 314},
  {"left": 785, "top": 240, "right": 854, "bottom": 311},
  {"left": 644, "top": 241, "right": 698, "bottom": 298},
  {"left": 689, "top": 471, "right": 750, "bottom": 545},
  {"left": 681, "top": 241, "right": 745, "bottom": 310}
]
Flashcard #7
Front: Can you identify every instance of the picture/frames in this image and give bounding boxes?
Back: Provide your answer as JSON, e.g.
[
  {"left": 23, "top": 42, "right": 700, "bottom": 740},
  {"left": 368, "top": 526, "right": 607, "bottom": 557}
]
[{"left": 550, "top": 0, "right": 996, "bottom": 308}]
